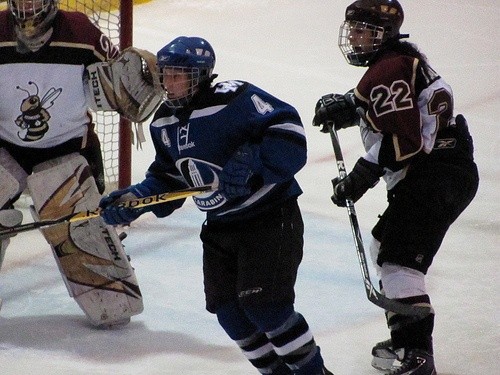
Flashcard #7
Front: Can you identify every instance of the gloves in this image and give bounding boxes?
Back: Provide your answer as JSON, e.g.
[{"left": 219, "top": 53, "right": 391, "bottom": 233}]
[
  {"left": 218, "top": 144, "right": 263, "bottom": 205},
  {"left": 99, "top": 177, "right": 164, "bottom": 225},
  {"left": 312, "top": 88, "right": 360, "bottom": 133},
  {"left": 331, "top": 157, "right": 386, "bottom": 207}
]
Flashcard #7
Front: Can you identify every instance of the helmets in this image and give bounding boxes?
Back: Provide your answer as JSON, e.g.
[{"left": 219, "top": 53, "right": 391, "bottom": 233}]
[
  {"left": 7, "top": 0, "right": 57, "bottom": 53},
  {"left": 152, "top": 36, "right": 215, "bottom": 108},
  {"left": 339, "top": 0, "right": 409, "bottom": 67}
]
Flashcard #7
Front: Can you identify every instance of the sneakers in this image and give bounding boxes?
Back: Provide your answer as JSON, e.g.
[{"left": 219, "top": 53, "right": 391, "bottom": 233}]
[
  {"left": 372, "top": 339, "right": 396, "bottom": 369},
  {"left": 384, "top": 349, "right": 436, "bottom": 375}
]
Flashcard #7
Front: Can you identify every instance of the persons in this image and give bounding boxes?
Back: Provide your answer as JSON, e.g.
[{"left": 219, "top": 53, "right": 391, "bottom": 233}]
[
  {"left": 98, "top": 36, "right": 332, "bottom": 375},
  {"left": 312, "top": 0, "right": 480, "bottom": 375},
  {"left": 0, "top": 0, "right": 163, "bottom": 329}
]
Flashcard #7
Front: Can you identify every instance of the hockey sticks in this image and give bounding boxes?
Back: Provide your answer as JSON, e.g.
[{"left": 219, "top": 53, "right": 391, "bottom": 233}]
[
  {"left": 0, "top": 182, "right": 219, "bottom": 237},
  {"left": 326, "top": 120, "right": 432, "bottom": 318}
]
